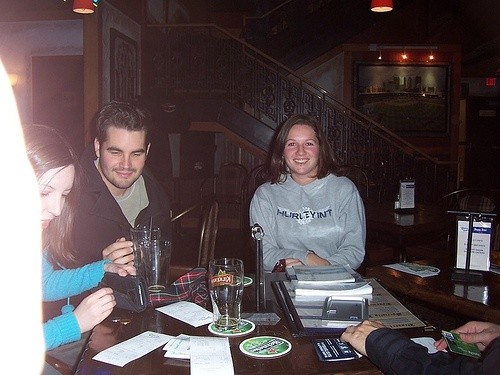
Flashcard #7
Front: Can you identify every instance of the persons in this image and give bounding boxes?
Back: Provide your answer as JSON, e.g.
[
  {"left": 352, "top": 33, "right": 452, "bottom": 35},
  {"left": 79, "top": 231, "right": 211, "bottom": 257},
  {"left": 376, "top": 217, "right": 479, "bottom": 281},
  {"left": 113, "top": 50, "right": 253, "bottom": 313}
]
[
  {"left": 249, "top": 114, "right": 365, "bottom": 274},
  {"left": 341, "top": 321, "right": 500, "bottom": 375},
  {"left": 20, "top": 101, "right": 174, "bottom": 355}
]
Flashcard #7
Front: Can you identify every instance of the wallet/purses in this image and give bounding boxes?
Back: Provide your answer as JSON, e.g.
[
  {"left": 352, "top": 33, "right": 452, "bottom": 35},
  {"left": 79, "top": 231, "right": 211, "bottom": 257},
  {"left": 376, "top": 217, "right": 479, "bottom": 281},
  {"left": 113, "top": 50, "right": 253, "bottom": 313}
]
[{"left": 90, "top": 271, "right": 151, "bottom": 313}]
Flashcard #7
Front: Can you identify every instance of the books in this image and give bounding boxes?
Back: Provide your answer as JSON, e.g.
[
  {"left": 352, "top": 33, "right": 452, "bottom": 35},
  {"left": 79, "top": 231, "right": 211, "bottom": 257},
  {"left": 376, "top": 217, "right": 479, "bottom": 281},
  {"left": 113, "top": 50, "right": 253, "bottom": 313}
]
[{"left": 285, "top": 264, "right": 372, "bottom": 296}]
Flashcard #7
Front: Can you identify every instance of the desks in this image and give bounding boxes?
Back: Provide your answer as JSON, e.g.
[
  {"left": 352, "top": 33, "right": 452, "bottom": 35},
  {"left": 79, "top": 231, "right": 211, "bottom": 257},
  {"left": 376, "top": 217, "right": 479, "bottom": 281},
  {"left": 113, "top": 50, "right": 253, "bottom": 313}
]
[
  {"left": 72, "top": 265, "right": 456, "bottom": 375},
  {"left": 365, "top": 200, "right": 456, "bottom": 263},
  {"left": 366, "top": 258, "right": 500, "bottom": 329}
]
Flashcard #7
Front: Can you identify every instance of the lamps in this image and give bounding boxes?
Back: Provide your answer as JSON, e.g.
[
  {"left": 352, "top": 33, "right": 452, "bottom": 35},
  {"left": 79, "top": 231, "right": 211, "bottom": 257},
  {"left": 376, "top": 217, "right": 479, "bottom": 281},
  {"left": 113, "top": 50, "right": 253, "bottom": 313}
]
[
  {"left": 371, "top": 0, "right": 393, "bottom": 12},
  {"left": 8, "top": 74, "right": 17, "bottom": 89},
  {"left": 72, "top": 0, "right": 94, "bottom": 14}
]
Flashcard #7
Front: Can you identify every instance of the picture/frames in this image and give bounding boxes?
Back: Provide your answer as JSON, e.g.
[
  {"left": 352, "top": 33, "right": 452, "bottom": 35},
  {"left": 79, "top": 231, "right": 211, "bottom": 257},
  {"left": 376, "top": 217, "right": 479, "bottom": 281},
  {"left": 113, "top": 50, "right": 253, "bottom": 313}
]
[
  {"left": 110, "top": 28, "right": 139, "bottom": 103},
  {"left": 352, "top": 59, "right": 451, "bottom": 136}
]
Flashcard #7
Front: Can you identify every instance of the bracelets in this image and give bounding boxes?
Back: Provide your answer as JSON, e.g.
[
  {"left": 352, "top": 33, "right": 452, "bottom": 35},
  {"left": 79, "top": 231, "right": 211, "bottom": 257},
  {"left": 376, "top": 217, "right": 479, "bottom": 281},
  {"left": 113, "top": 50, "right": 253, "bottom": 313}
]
[{"left": 307, "top": 250, "right": 314, "bottom": 254}]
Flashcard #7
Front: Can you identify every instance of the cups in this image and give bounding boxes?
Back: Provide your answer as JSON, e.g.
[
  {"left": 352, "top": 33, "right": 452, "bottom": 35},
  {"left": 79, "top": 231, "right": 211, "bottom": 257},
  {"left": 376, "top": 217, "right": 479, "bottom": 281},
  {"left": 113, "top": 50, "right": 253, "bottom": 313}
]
[
  {"left": 139, "top": 239, "right": 172, "bottom": 288},
  {"left": 208, "top": 257, "right": 244, "bottom": 330},
  {"left": 130, "top": 226, "right": 161, "bottom": 275}
]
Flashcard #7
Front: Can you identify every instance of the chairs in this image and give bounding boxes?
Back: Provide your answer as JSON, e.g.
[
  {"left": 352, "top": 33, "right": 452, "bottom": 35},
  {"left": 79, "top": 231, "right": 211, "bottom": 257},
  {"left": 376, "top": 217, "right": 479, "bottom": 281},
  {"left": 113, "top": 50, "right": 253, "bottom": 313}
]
[{"left": 171, "top": 163, "right": 500, "bottom": 269}]
